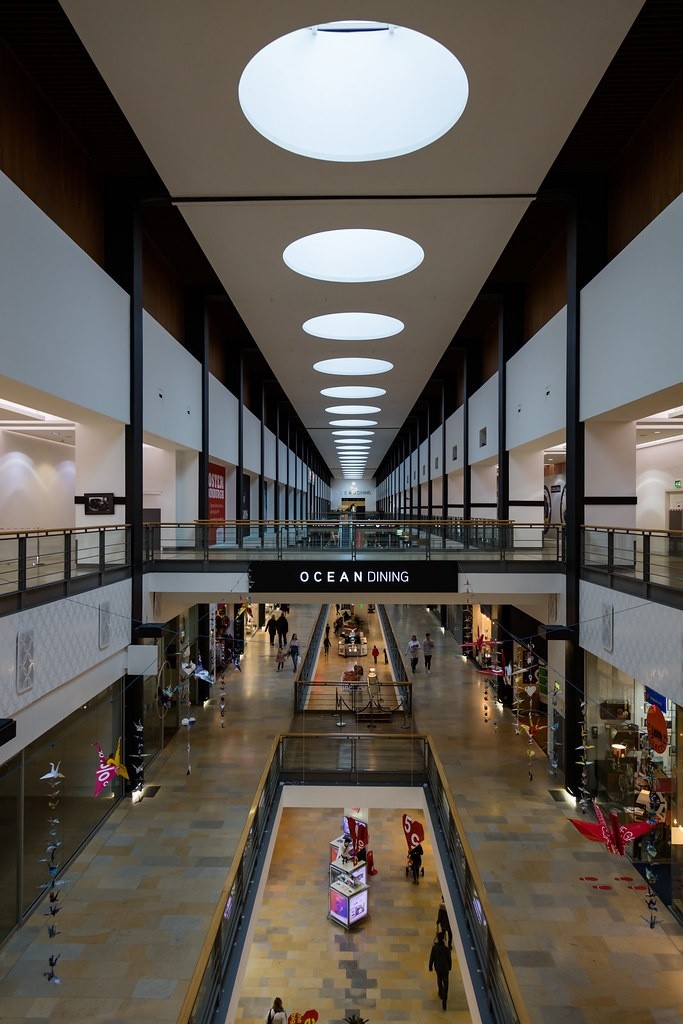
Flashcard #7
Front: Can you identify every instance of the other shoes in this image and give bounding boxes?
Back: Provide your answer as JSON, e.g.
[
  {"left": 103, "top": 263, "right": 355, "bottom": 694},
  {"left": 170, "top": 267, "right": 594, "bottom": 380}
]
[
  {"left": 417, "top": 882, "right": 420, "bottom": 885},
  {"left": 412, "top": 880, "right": 416, "bottom": 885},
  {"left": 281, "top": 669, "right": 284, "bottom": 671},
  {"left": 427, "top": 670, "right": 431, "bottom": 674},
  {"left": 424, "top": 666, "right": 427, "bottom": 672},
  {"left": 277, "top": 670, "right": 280, "bottom": 672},
  {"left": 293, "top": 667, "right": 297, "bottom": 673}
]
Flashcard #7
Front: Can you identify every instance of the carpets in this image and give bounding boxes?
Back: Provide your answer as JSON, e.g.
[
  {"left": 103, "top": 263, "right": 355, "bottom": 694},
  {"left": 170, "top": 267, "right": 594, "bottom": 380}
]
[
  {"left": 125, "top": 784, "right": 162, "bottom": 798},
  {"left": 548, "top": 788, "right": 576, "bottom": 802}
]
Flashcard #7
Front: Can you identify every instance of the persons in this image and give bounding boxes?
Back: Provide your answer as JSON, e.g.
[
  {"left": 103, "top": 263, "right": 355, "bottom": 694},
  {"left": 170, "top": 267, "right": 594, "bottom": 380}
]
[
  {"left": 279, "top": 603, "right": 290, "bottom": 617},
  {"left": 405, "top": 634, "right": 421, "bottom": 674},
  {"left": 371, "top": 645, "right": 379, "bottom": 664},
  {"left": 275, "top": 647, "right": 286, "bottom": 672},
  {"left": 277, "top": 613, "right": 288, "bottom": 650},
  {"left": 326, "top": 624, "right": 330, "bottom": 636},
  {"left": 264, "top": 996, "right": 287, "bottom": 1024},
  {"left": 411, "top": 843, "right": 424, "bottom": 885},
  {"left": 323, "top": 636, "right": 331, "bottom": 656},
  {"left": 429, "top": 895, "right": 452, "bottom": 1012},
  {"left": 287, "top": 633, "right": 300, "bottom": 673},
  {"left": 336, "top": 838, "right": 351, "bottom": 860},
  {"left": 335, "top": 604, "right": 340, "bottom": 615},
  {"left": 422, "top": 633, "right": 435, "bottom": 674},
  {"left": 265, "top": 614, "right": 276, "bottom": 647}
]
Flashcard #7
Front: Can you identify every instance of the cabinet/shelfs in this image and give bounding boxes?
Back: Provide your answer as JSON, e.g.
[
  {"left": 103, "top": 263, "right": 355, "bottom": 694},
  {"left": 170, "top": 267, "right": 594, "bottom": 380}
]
[{"left": 606, "top": 726, "right": 640, "bottom": 791}]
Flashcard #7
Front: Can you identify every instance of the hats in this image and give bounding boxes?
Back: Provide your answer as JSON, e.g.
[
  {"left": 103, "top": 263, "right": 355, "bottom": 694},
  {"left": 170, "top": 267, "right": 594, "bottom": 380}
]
[{"left": 411, "top": 633, "right": 417, "bottom": 640}]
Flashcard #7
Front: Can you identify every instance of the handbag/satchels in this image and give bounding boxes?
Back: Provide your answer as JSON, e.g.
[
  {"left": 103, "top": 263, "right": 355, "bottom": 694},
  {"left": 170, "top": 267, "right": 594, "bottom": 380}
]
[
  {"left": 268, "top": 1009, "right": 272, "bottom": 1024},
  {"left": 333, "top": 621, "right": 337, "bottom": 627},
  {"left": 287, "top": 607, "right": 289, "bottom": 613}
]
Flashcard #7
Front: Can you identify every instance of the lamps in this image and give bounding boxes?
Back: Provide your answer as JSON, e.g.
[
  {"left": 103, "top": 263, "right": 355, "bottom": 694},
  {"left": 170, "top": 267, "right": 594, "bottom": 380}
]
[
  {"left": 133, "top": 622, "right": 170, "bottom": 638},
  {"left": 538, "top": 625, "right": 575, "bottom": 641}
]
